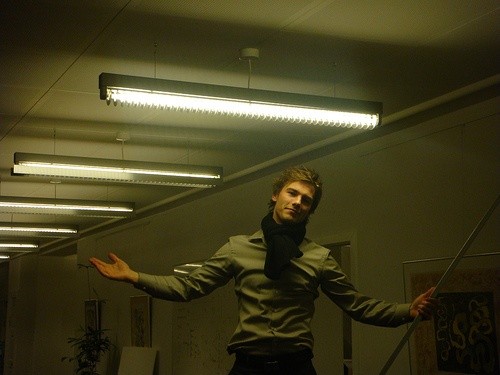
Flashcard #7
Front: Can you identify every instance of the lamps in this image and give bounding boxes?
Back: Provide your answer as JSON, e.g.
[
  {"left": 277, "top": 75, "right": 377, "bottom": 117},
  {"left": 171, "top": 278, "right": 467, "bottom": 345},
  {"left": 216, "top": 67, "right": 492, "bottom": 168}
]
[
  {"left": 13, "top": 131, "right": 223, "bottom": 190},
  {"left": 0, "top": 213, "right": 78, "bottom": 236},
  {"left": 98, "top": 47, "right": 384, "bottom": 130},
  {"left": 0, "top": 179, "right": 135, "bottom": 219},
  {"left": 0, "top": 240, "right": 39, "bottom": 260}
]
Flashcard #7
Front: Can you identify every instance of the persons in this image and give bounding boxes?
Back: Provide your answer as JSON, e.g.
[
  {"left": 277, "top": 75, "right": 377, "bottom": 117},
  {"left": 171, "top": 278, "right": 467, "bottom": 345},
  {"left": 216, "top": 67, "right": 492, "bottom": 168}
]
[{"left": 88, "top": 165, "right": 440, "bottom": 375}]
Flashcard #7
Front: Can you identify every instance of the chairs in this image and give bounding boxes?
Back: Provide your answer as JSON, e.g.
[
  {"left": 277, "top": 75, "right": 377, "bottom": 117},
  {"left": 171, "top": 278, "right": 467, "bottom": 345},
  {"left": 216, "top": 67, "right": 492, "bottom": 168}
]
[{"left": 118, "top": 346, "right": 159, "bottom": 375}]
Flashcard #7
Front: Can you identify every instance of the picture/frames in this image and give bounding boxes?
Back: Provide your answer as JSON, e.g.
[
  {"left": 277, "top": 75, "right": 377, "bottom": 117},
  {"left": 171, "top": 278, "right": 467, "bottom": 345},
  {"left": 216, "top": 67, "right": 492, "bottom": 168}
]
[
  {"left": 128, "top": 295, "right": 152, "bottom": 347},
  {"left": 83, "top": 299, "right": 101, "bottom": 355},
  {"left": 401, "top": 252, "right": 500, "bottom": 375}
]
